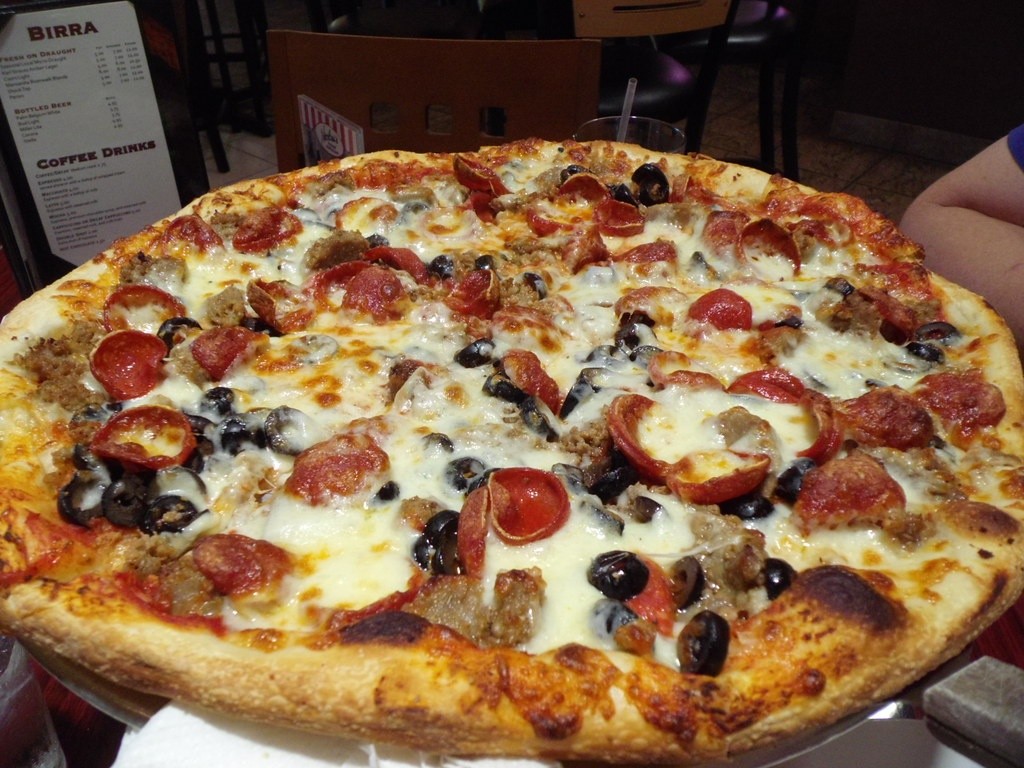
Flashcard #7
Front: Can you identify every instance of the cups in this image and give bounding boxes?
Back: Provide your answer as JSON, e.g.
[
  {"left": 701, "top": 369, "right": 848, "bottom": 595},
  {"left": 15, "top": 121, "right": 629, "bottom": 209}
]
[{"left": 572, "top": 115, "right": 686, "bottom": 158}]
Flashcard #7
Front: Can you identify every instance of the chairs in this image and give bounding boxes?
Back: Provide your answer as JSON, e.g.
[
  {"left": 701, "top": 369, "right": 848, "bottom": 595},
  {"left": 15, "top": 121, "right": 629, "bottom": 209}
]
[
  {"left": 267, "top": 28, "right": 601, "bottom": 172},
  {"left": 567, "top": 0, "right": 803, "bottom": 183}
]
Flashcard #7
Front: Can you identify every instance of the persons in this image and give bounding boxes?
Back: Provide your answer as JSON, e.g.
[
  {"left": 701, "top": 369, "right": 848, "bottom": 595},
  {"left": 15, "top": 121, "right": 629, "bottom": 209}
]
[{"left": 899, "top": 122, "right": 1024, "bottom": 363}]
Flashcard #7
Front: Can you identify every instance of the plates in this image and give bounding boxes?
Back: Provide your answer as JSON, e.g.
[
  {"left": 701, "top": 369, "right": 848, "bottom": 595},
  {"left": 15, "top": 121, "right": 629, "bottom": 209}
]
[{"left": 12, "top": 632, "right": 890, "bottom": 768}]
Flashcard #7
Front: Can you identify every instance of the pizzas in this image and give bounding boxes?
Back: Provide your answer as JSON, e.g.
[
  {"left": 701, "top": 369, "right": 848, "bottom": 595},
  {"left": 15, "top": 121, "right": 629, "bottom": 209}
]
[{"left": 0, "top": 136, "right": 1023, "bottom": 768}]
